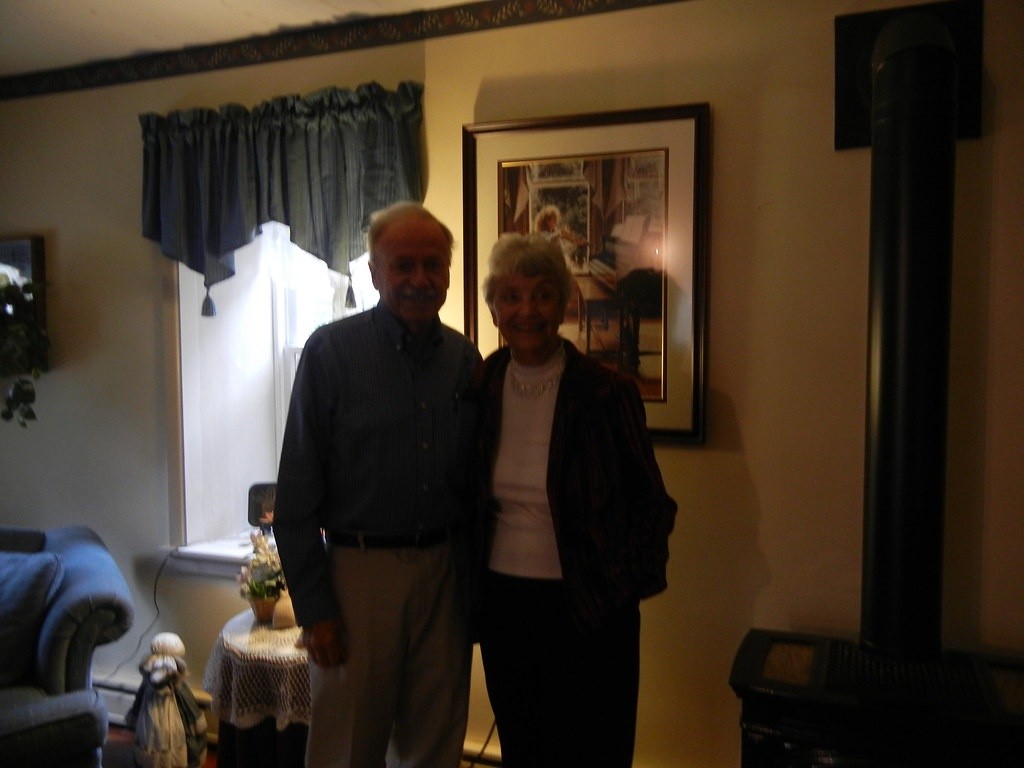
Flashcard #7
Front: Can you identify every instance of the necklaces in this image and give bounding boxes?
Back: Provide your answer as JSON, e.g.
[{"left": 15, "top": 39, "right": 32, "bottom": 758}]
[{"left": 507, "top": 354, "right": 567, "bottom": 401}]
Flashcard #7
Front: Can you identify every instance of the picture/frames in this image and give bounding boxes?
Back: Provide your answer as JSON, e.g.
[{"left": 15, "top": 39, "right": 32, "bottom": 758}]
[{"left": 458, "top": 100, "right": 721, "bottom": 440}]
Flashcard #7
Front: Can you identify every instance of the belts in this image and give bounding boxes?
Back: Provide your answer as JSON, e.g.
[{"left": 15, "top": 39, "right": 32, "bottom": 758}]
[{"left": 322, "top": 516, "right": 478, "bottom": 550}]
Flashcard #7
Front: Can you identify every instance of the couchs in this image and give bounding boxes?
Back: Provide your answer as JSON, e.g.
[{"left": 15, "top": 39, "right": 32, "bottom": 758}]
[{"left": 0, "top": 523, "right": 138, "bottom": 768}]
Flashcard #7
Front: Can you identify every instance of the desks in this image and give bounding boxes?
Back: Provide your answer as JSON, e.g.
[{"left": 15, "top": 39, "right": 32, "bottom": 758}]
[{"left": 207, "top": 600, "right": 315, "bottom": 768}]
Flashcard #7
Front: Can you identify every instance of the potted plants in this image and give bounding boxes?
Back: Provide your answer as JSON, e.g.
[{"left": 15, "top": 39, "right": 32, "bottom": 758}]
[{"left": 242, "top": 531, "right": 290, "bottom": 623}]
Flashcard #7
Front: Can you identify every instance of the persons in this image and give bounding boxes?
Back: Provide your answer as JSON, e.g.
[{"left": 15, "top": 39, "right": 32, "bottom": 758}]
[
  {"left": 474, "top": 228, "right": 677, "bottom": 768},
  {"left": 270, "top": 201, "right": 489, "bottom": 768}
]
